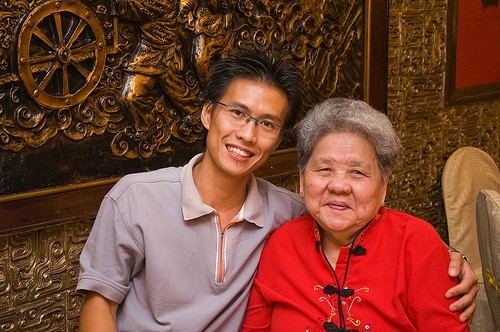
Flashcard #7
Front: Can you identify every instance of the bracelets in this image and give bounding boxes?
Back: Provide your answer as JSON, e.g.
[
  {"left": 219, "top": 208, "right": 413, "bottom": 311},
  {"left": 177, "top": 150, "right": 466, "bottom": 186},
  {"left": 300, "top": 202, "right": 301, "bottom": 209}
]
[{"left": 448, "top": 247, "right": 470, "bottom": 264}]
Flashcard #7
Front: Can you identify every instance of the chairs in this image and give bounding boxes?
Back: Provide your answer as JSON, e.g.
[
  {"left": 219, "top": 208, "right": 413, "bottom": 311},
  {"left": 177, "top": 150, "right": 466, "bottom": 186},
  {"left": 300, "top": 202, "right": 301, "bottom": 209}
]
[{"left": 440, "top": 146, "right": 500, "bottom": 332}]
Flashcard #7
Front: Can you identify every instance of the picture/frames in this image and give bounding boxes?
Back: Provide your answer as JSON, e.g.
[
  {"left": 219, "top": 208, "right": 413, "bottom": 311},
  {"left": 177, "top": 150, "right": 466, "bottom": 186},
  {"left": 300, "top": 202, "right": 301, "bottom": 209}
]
[
  {"left": 444, "top": 0, "right": 500, "bottom": 107},
  {"left": 0, "top": 0, "right": 388, "bottom": 239}
]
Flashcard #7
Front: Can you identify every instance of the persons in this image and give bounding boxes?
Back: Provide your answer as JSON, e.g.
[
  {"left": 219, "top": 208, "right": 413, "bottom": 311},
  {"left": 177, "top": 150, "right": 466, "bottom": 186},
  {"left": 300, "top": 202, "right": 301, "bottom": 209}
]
[
  {"left": 113, "top": 0, "right": 241, "bottom": 143},
  {"left": 241, "top": 97, "right": 470, "bottom": 332},
  {"left": 75, "top": 50, "right": 481, "bottom": 332}
]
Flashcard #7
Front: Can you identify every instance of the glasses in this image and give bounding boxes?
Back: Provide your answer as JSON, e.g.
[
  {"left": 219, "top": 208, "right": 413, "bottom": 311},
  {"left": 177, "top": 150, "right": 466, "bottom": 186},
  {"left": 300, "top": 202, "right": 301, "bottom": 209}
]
[{"left": 213, "top": 101, "right": 283, "bottom": 136}]
